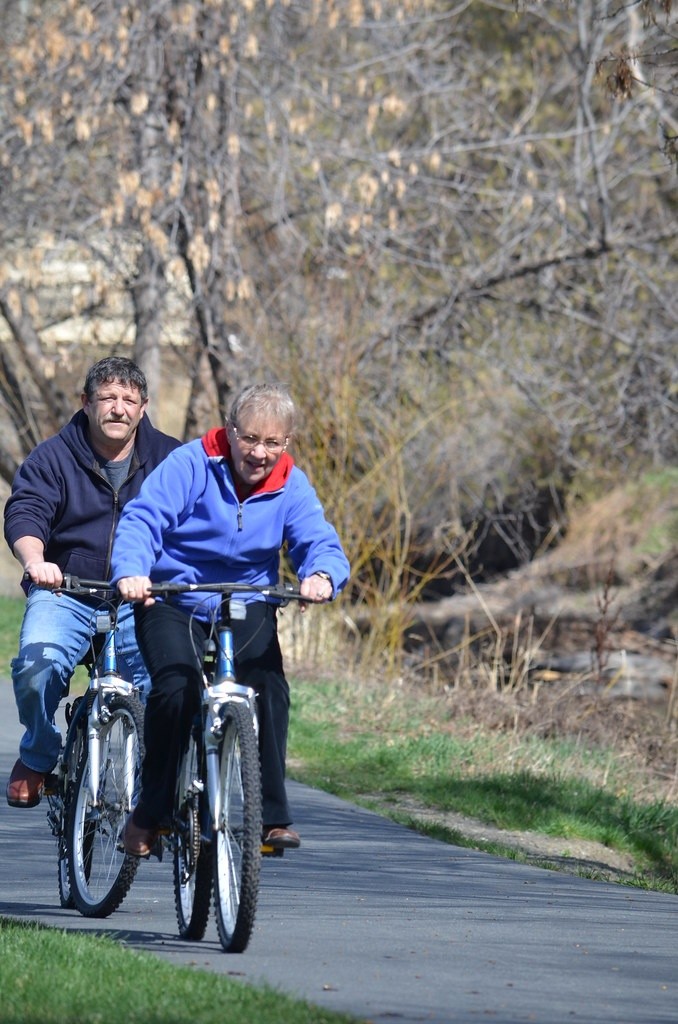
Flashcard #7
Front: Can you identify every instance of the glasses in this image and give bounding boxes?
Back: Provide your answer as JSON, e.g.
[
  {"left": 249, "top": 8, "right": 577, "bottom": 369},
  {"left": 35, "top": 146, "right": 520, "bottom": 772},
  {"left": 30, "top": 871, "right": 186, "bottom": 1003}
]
[{"left": 232, "top": 424, "right": 287, "bottom": 452}]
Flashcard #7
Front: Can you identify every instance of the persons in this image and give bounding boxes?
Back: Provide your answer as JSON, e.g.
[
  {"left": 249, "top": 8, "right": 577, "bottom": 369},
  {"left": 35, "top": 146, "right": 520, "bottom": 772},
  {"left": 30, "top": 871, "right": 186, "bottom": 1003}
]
[
  {"left": 3, "top": 357, "right": 183, "bottom": 809},
  {"left": 111, "top": 384, "right": 354, "bottom": 858}
]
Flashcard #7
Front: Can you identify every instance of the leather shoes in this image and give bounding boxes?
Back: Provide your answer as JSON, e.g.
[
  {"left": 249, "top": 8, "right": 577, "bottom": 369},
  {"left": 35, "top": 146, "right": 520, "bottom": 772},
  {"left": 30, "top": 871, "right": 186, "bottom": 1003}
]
[
  {"left": 6, "top": 757, "right": 49, "bottom": 808},
  {"left": 122, "top": 813, "right": 154, "bottom": 857},
  {"left": 260, "top": 823, "right": 301, "bottom": 848}
]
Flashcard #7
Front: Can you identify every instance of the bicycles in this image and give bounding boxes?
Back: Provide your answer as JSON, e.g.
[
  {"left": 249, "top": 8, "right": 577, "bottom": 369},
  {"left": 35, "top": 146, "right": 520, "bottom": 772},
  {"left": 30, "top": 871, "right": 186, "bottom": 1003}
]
[
  {"left": 17, "top": 571, "right": 147, "bottom": 920},
  {"left": 118, "top": 582, "right": 335, "bottom": 955}
]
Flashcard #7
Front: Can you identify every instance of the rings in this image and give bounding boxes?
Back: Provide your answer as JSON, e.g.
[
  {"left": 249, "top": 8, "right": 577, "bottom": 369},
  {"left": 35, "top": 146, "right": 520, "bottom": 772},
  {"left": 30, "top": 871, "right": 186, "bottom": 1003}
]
[
  {"left": 129, "top": 587, "right": 135, "bottom": 592},
  {"left": 318, "top": 591, "right": 325, "bottom": 597}
]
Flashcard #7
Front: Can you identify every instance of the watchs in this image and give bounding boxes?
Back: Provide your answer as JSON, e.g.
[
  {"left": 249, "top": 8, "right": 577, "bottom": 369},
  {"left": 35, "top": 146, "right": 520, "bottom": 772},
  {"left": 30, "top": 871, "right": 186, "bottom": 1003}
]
[{"left": 317, "top": 571, "right": 331, "bottom": 580}]
